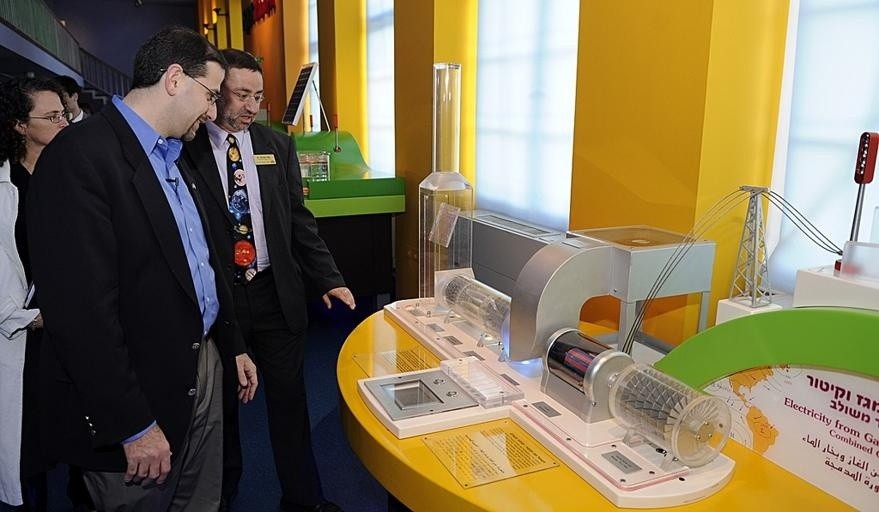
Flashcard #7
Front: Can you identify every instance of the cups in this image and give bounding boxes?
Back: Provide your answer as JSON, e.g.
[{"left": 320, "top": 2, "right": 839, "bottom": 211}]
[
  {"left": 27, "top": 111, "right": 73, "bottom": 124},
  {"left": 224, "top": 84, "right": 266, "bottom": 103},
  {"left": 159, "top": 65, "right": 224, "bottom": 106}
]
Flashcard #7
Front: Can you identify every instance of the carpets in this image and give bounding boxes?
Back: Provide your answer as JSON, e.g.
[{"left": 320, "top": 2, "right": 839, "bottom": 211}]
[{"left": 225, "top": 133, "right": 259, "bottom": 287}]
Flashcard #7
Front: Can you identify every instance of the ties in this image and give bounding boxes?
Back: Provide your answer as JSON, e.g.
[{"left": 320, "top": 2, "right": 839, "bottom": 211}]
[{"left": 281, "top": 62, "right": 318, "bottom": 127}]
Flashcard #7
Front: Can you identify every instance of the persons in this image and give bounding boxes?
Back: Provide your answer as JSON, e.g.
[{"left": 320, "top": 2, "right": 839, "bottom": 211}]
[
  {"left": 189, "top": 47, "right": 356, "bottom": 512},
  {"left": 1, "top": 73, "right": 73, "bottom": 512},
  {"left": 31, "top": 24, "right": 259, "bottom": 512},
  {"left": 58, "top": 74, "right": 89, "bottom": 126}
]
